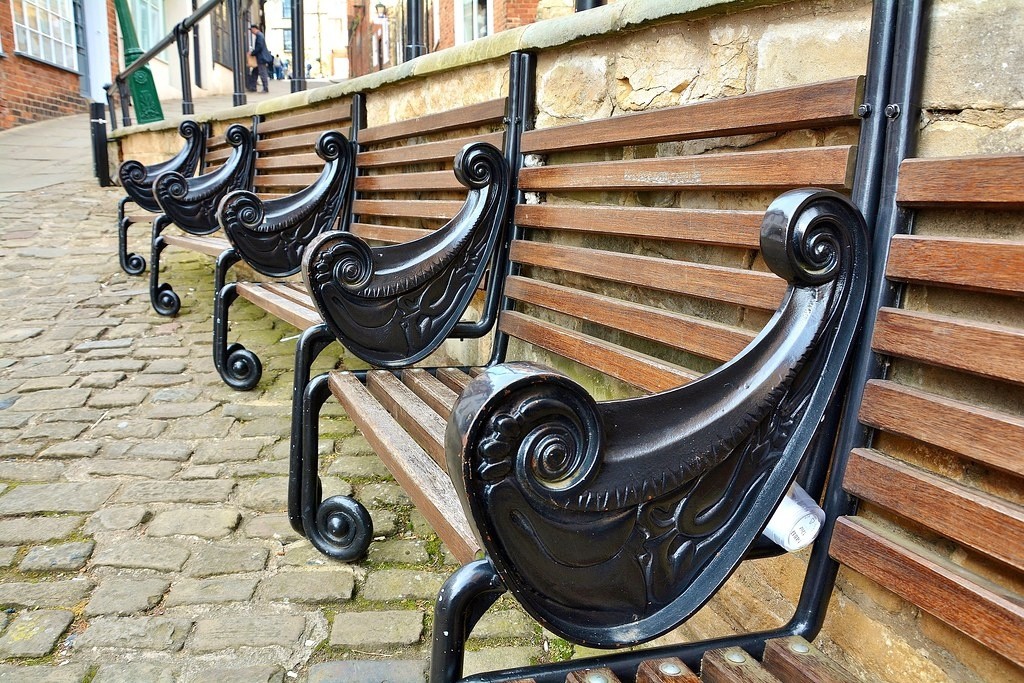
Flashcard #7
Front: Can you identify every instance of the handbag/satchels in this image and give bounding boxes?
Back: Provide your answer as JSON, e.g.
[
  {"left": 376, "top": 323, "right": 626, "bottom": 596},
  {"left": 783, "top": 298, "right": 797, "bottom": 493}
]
[{"left": 246, "top": 52, "right": 257, "bottom": 68}]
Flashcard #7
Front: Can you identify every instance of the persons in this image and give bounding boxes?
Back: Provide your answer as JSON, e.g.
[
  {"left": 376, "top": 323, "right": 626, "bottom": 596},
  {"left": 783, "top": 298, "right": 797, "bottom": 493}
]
[{"left": 248, "top": 23, "right": 292, "bottom": 93}]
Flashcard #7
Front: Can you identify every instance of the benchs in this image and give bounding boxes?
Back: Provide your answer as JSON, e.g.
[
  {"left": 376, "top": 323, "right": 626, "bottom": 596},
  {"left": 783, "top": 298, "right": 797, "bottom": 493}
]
[{"left": 117, "top": 1, "right": 1024, "bottom": 682}]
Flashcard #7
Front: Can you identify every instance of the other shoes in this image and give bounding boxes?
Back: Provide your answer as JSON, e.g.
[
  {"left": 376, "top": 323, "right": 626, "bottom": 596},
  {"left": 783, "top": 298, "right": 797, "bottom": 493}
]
[
  {"left": 247, "top": 89, "right": 256, "bottom": 92},
  {"left": 261, "top": 90, "right": 268, "bottom": 93}
]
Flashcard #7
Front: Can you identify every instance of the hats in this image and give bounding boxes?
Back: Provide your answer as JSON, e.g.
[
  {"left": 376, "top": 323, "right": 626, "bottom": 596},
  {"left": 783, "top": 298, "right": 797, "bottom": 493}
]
[{"left": 249, "top": 25, "right": 257, "bottom": 30}]
